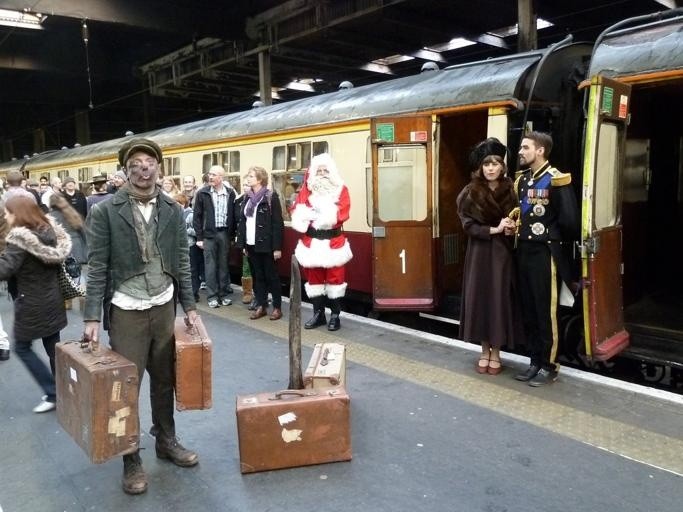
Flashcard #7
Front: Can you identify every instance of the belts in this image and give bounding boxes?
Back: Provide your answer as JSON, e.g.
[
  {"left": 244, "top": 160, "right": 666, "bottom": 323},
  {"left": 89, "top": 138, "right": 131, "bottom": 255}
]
[{"left": 307, "top": 227, "right": 341, "bottom": 239}]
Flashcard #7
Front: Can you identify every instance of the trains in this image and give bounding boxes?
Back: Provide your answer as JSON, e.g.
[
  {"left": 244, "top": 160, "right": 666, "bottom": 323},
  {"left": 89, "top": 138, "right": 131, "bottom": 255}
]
[{"left": 0, "top": 7, "right": 683, "bottom": 387}]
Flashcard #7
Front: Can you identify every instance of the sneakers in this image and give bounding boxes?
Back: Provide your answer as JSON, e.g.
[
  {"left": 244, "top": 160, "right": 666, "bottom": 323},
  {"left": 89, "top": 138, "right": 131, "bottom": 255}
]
[
  {"left": 221, "top": 298, "right": 233, "bottom": 305},
  {"left": 208, "top": 299, "right": 219, "bottom": 308},
  {"left": 200, "top": 282, "right": 207, "bottom": 289}
]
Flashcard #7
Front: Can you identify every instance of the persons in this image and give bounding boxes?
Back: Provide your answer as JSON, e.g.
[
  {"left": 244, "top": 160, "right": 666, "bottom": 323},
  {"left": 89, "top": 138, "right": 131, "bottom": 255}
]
[
  {"left": 1, "top": 190, "right": 68, "bottom": 413},
  {"left": 161, "top": 164, "right": 283, "bottom": 320},
  {"left": 455, "top": 136, "right": 522, "bottom": 375},
  {"left": 1, "top": 329, "right": 9, "bottom": 359},
  {"left": 291, "top": 150, "right": 352, "bottom": 330},
  {"left": 1, "top": 171, "right": 127, "bottom": 312},
  {"left": 504, "top": 128, "right": 583, "bottom": 388},
  {"left": 82, "top": 141, "right": 198, "bottom": 494}
]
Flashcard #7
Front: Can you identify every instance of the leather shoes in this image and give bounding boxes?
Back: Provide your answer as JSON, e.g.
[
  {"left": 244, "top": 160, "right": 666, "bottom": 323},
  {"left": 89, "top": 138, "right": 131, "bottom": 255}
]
[
  {"left": 33, "top": 400, "right": 56, "bottom": 413},
  {"left": 248, "top": 299, "right": 282, "bottom": 319},
  {"left": 123, "top": 451, "right": 148, "bottom": 493},
  {"left": 156, "top": 438, "right": 199, "bottom": 466},
  {"left": 514, "top": 363, "right": 538, "bottom": 381},
  {"left": 1, "top": 349, "right": 10, "bottom": 360},
  {"left": 528, "top": 368, "right": 558, "bottom": 387}
]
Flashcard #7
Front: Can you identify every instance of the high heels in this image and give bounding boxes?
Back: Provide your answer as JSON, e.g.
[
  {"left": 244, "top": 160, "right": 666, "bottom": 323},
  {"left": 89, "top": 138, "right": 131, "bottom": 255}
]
[
  {"left": 477, "top": 351, "right": 490, "bottom": 374},
  {"left": 488, "top": 354, "right": 502, "bottom": 375}
]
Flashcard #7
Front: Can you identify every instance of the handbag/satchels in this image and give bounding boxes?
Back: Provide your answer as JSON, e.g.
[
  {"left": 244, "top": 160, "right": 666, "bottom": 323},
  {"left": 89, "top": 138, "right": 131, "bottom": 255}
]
[
  {"left": 59, "top": 263, "right": 86, "bottom": 299},
  {"left": 65, "top": 256, "right": 83, "bottom": 285}
]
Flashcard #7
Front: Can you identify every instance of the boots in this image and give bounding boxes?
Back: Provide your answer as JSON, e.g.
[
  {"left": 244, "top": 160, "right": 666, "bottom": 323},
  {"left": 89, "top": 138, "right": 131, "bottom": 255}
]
[
  {"left": 328, "top": 298, "right": 341, "bottom": 330},
  {"left": 241, "top": 277, "right": 253, "bottom": 303},
  {"left": 305, "top": 294, "right": 326, "bottom": 329}
]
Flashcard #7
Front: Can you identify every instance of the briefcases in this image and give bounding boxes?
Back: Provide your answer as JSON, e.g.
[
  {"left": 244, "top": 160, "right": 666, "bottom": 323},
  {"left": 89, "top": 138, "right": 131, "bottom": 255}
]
[
  {"left": 304, "top": 343, "right": 347, "bottom": 389},
  {"left": 55, "top": 342, "right": 140, "bottom": 464},
  {"left": 235, "top": 385, "right": 352, "bottom": 473},
  {"left": 174, "top": 315, "right": 212, "bottom": 412}
]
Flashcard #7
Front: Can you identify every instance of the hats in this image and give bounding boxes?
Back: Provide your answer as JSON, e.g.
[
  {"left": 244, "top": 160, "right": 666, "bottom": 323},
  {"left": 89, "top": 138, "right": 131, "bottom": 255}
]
[
  {"left": 116, "top": 170, "right": 127, "bottom": 183},
  {"left": 467, "top": 138, "right": 506, "bottom": 172},
  {"left": 118, "top": 138, "right": 163, "bottom": 165},
  {"left": 63, "top": 178, "right": 76, "bottom": 187},
  {"left": 91, "top": 176, "right": 110, "bottom": 183}
]
